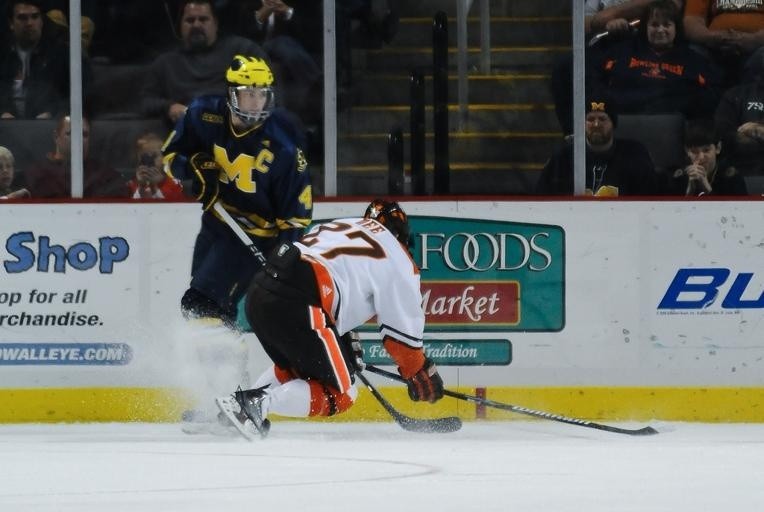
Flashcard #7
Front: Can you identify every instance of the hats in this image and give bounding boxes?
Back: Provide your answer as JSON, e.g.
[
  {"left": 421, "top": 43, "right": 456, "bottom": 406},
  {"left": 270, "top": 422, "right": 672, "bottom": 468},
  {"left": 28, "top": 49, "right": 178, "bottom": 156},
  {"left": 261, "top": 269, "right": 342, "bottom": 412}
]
[{"left": 585, "top": 95, "right": 618, "bottom": 125}]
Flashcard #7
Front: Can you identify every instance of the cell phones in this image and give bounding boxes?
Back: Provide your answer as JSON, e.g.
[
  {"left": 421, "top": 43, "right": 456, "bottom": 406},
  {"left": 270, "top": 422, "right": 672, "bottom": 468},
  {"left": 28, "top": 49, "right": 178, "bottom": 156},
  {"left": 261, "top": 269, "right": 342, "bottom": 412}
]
[{"left": 141, "top": 152, "right": 154, "bottom": 167}]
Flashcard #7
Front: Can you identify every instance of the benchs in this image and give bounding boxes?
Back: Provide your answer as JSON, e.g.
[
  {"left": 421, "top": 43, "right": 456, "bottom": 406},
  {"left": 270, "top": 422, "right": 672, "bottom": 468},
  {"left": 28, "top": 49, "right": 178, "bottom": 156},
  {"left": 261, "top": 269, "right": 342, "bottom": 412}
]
[{"left": 0, "top": 0, "right": 764, "bottom": 195}]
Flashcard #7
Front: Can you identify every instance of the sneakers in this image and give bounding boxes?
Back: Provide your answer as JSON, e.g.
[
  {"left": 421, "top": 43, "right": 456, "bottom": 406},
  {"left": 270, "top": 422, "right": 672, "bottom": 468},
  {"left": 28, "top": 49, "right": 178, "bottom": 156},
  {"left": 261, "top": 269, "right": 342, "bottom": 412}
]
[{"left": 231, "top": 389, "right": 271, "bottom": 440}]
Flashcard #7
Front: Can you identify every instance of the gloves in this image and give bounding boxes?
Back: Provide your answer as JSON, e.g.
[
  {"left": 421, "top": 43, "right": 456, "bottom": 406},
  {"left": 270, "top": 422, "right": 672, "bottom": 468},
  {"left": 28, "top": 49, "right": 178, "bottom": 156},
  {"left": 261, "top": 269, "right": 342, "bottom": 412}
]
[
  {"left": 398, "top": 355, "right": 443, "bottom": 404},
  {"left": 186, "top": 154, "right": 220, "bottom": 211}
]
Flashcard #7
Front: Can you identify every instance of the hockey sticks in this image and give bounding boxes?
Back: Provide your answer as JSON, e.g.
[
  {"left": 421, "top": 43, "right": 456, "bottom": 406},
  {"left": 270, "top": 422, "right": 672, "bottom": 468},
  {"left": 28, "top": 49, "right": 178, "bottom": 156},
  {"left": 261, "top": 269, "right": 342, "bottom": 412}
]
[
  {"left": 360, "top": 363, "right": 658, "bottom": 435},
  {"left": 213, "top": 202, "right": 461, "bottom": 433}
]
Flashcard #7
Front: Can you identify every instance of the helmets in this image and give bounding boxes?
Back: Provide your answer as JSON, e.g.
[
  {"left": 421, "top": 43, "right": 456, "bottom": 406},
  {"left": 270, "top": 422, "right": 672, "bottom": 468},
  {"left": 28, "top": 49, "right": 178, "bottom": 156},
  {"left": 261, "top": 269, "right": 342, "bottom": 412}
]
[
  {"left": 364, "top": 199, "right": 408, "bottom": 244},
  {"left": 223, "top": 54, "right": 275, "bottom": 125}
]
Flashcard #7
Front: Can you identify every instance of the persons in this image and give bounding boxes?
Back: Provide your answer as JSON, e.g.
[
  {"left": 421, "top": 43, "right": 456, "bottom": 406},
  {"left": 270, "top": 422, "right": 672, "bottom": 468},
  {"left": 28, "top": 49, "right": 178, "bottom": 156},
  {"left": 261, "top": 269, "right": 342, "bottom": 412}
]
[
  {"left": 538, "top": 1, "right": 762, "bottom": 199},
  {"left": 159, "top": 54, "right": 314, "bottom": 429},
  {"left": 230, "top": 199, "right": 444, "bottom": 440},
  {"left": 1, "top": 1, "right": 403, "bottom": 199}
]
